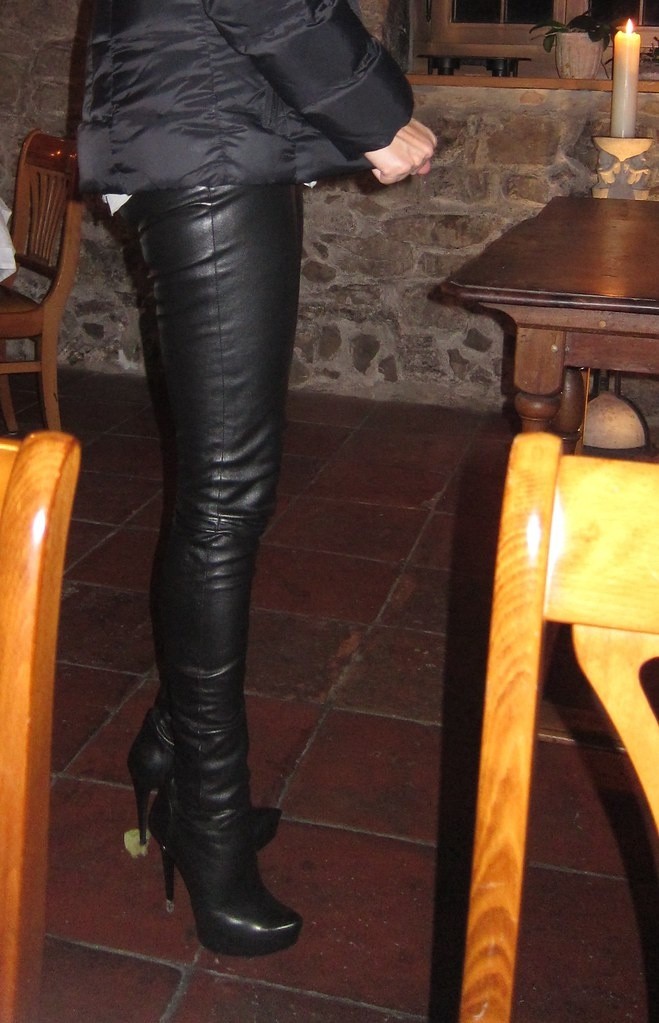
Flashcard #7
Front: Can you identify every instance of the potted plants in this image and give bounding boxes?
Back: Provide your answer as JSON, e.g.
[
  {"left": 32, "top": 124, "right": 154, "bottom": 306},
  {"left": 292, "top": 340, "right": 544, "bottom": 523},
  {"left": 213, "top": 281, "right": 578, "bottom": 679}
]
[{"left": 530, "top": 4, "right": 617, "bottom": 79}]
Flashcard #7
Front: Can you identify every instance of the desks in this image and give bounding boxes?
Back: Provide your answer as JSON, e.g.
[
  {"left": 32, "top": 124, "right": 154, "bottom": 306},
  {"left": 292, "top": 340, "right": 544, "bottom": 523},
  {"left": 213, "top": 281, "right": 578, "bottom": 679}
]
[{"left": 434, "top": 194, "right": 659, "bottom": 463}]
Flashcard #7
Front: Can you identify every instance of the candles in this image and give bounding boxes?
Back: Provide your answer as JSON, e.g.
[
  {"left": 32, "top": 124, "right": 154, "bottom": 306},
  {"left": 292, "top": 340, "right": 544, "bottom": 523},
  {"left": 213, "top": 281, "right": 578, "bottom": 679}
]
[{"left": 610, "top": 17, "right": 641, "bottom": 138}]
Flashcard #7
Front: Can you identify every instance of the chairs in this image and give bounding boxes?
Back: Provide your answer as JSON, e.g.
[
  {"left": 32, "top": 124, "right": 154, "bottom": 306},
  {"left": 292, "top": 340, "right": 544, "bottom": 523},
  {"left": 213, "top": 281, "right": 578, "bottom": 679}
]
[
  {"left": 459, "top": 433, "right": 659, "bottom": 1023},
  {"left": 0, "top": 128, "right": 87, "bottom": 436}
]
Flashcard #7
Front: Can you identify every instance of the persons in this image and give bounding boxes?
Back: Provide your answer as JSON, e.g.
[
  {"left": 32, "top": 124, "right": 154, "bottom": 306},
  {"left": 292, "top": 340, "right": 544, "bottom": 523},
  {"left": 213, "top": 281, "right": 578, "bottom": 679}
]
[{"left": 71, "top": 0, "right": 436, "bottom": 956}]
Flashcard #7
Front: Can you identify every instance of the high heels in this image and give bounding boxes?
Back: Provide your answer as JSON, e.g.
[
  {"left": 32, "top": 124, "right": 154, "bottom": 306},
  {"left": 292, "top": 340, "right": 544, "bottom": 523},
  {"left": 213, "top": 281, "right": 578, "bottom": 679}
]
[
  {"left": 148, "top": 774, "right": 301, "bottom": 955},
  {"left": 128, "top": 709, "right": 281, "bottom": 859}
]
